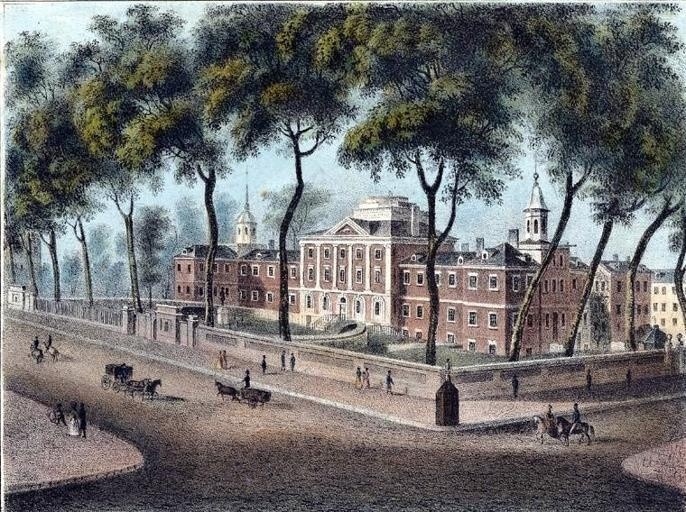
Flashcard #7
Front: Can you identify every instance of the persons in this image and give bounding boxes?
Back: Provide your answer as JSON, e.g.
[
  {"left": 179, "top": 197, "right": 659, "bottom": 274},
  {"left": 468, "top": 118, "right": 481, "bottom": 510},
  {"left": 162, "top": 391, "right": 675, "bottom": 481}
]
[
  {"left": 31, "top": 337, "right": 39, "bottom": 356},
  {"left": 218, "top": 349, "right": 295, "bottom": 390},
  {"left": 587, "top": 369, "right": 591, "bottom": 391},
  {"left": 512, "top": 373, "right": 518, "bottom": 396},
  {"left": 570, "top": 403, "right": 582, "bottom": 434},
  {"left": 383, "top": 370, "right": 394, "bottom": 396},
  {"left": 626, "top": 369, "right": 632, "bottom": 388},
  {"left": 352, "top": 367, "right": 370, "bottom": 393},
  {"left": 546, "top": 404, "right": 554, "bottom": 421},
  {"left": 54, "top": 401, "right": 87, "bottom": 438},
  {"left": 46, "top": 335, "right": 52, "bottom": 352}
]
